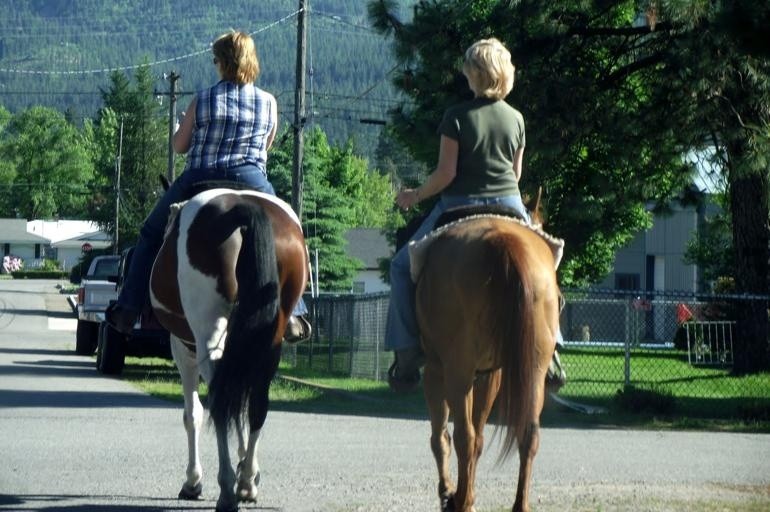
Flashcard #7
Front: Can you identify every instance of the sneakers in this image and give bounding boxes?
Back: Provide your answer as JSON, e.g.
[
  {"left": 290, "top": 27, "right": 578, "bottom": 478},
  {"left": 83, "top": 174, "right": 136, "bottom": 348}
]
[
  {"left": 547, "top": 356, "right": 560, "bottom": 383},
  {"left": 110, "top": 304, "right": 136, "bottom": 333},
  {"left": 395, "top": 344, "right": 425, "bottom": 381},
  {"left": 283, "top": 316, "right": 306, "bottom": 343}
]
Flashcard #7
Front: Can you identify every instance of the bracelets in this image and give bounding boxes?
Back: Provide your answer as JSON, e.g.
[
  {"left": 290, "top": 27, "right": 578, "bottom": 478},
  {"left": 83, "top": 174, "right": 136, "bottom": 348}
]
[{"left": 414, "top": 186, "right": 422, "bottom": 203}]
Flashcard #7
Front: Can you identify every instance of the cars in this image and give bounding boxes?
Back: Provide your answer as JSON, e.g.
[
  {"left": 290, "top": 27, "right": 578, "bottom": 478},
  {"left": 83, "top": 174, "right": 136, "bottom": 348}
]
[{"left": 75, "top": 255, "right": 173, "bottom": 375}]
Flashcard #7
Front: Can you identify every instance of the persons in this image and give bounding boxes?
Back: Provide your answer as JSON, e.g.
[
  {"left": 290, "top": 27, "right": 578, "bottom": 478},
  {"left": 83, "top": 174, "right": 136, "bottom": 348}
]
[
  {"left": 2, "top": 254, "right": 24, "bottom": 274},
  {"left": 383, "top": 36, "right": 566, "bottom": 400},
  {"left": 105, "top": 32, "right": 312, "bottom": 344}
]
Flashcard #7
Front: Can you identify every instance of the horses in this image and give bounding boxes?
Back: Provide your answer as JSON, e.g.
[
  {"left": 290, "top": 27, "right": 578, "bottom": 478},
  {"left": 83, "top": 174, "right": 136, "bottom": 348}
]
[
  {"left": 147, "top": 174, "right": 310, "bottom": 512},
  {"left": 413, "top": 185, "right": 564, "bottom": 512}
]
